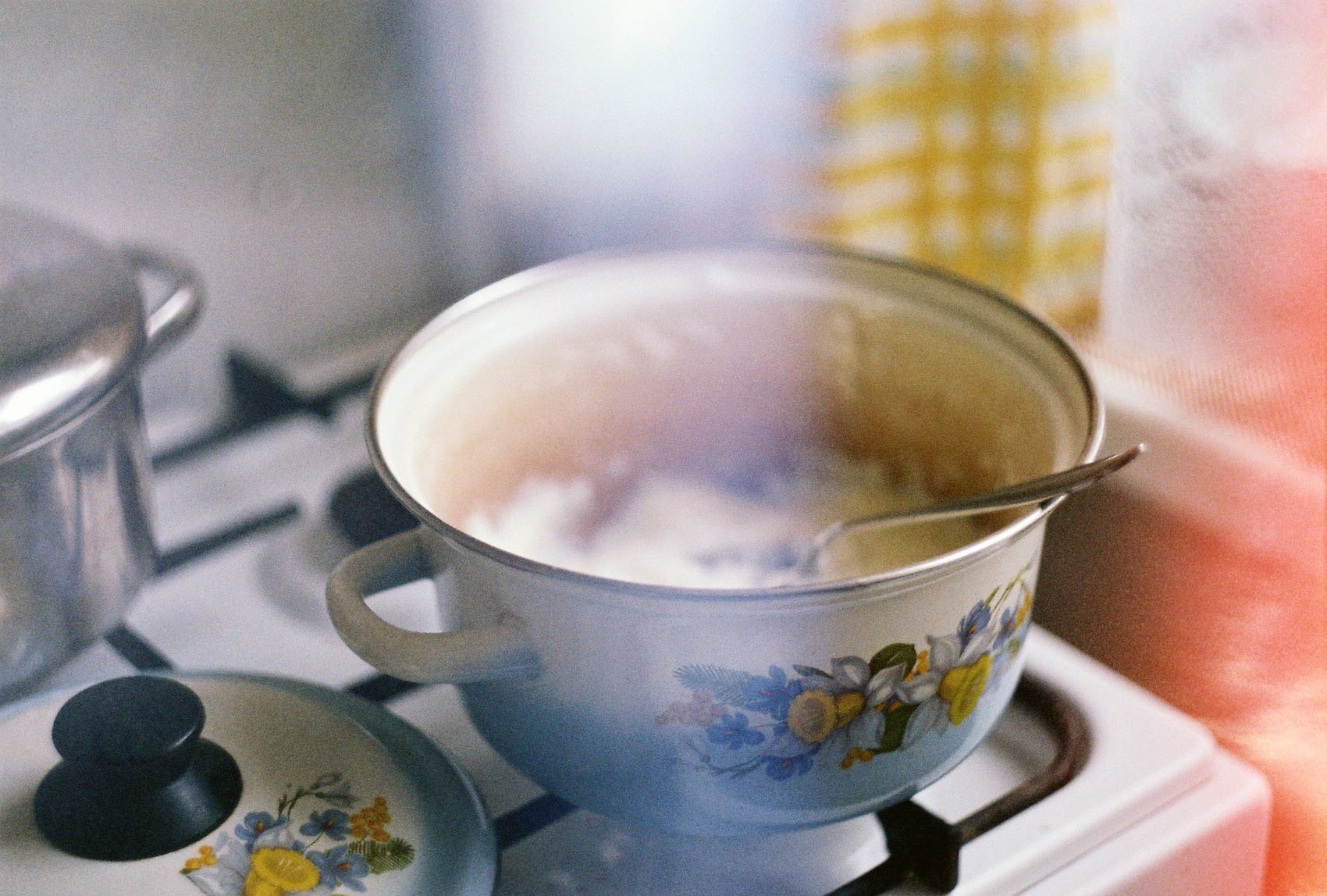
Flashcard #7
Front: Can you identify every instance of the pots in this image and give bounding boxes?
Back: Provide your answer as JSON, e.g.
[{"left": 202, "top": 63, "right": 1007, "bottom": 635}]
[
  {"left": 325, "top": 233, "right": 1106, "bottom": 840},
  {"left": 0, "top": 205, "right": 208, "bottom": 707}
]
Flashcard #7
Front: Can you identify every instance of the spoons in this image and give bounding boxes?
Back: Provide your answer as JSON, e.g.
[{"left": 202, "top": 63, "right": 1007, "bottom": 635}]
[{"left": 693, "top": 444, "right": 1148, "bottom": 586}]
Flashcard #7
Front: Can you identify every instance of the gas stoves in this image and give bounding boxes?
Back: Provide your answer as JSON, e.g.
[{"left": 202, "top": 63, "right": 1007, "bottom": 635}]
[{"left": 0, "top": 311, "right": 1274, "bottom": 896}]
